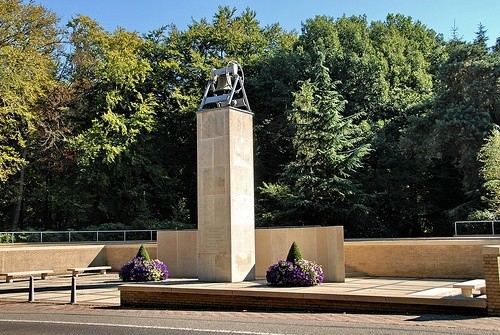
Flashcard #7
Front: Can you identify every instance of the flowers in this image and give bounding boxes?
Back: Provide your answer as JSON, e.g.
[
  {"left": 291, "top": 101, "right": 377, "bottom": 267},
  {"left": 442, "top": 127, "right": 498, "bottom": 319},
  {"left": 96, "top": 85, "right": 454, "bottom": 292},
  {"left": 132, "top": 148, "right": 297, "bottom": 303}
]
[
  {"left": 118, "top": 257, "right": 168, "bottom": 281},
  {"left": 265, "top": 259, "right": 324, "bottom": 286}
]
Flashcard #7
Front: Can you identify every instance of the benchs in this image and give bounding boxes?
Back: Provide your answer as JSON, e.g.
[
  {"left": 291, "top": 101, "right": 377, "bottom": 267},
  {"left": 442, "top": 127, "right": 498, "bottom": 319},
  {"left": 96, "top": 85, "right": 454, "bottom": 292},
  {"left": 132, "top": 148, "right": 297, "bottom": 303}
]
[
  {"left": 67, "top": 265, "right": 112, "bottom": 277},
  {"left": 452, "top": 278, "right": 486, "bottom": 299},
  {"left": 0, "top": 269, "right": 54, "bottom": 283}
]
